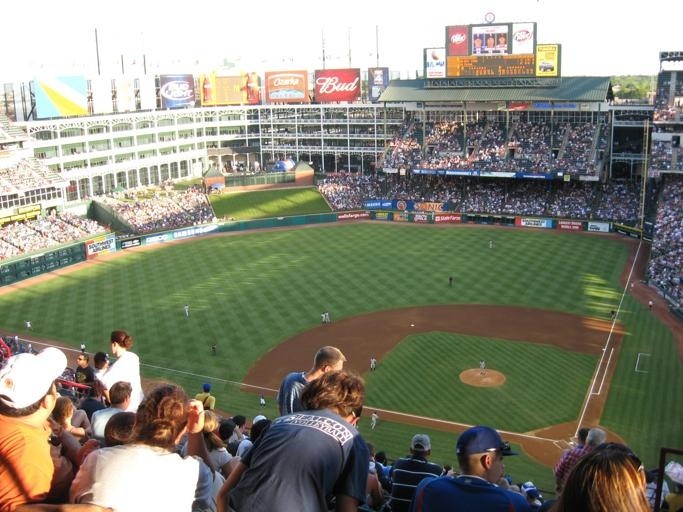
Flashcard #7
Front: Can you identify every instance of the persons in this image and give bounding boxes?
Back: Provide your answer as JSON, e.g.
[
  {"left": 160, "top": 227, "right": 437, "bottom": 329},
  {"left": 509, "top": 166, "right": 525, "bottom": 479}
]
[
  {"left": 1, "top": 106, "right": 683, "bottom": 327},
  {"left": 485, "top": 37, "right": 495, "bottom": 49},
  {"left": 474, "top": 37, "right": 485, "bottom": 53},
  {"left": 1, "top": 330, "right": 682, "bottom": 511},
  {"left": 496, "top": 37, "right": 507, "bottom": 48}
]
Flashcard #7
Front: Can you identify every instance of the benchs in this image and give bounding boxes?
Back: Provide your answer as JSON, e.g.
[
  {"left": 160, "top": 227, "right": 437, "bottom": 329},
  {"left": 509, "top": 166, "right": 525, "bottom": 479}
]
[
  {"left": 374, "top": 120, "right": 640, "bottom": 221},
  {"left": 0, "top": 111, "right": 64, "bottom": 184}
]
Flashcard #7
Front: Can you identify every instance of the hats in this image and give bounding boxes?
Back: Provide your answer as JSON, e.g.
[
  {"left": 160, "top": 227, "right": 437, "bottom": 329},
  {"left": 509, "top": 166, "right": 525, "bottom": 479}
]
[
  {"left": 203, "top": 383, "right": 211, "bottom": 391},
  {"left": 456, "top": 425, "right": 518, "bottom": 455},
  {"left": 0, "top": 348, "right": 67, "bottom": 409},
  {"left": 411, "top": 434, "right": 430, "bottom": 451}
]
